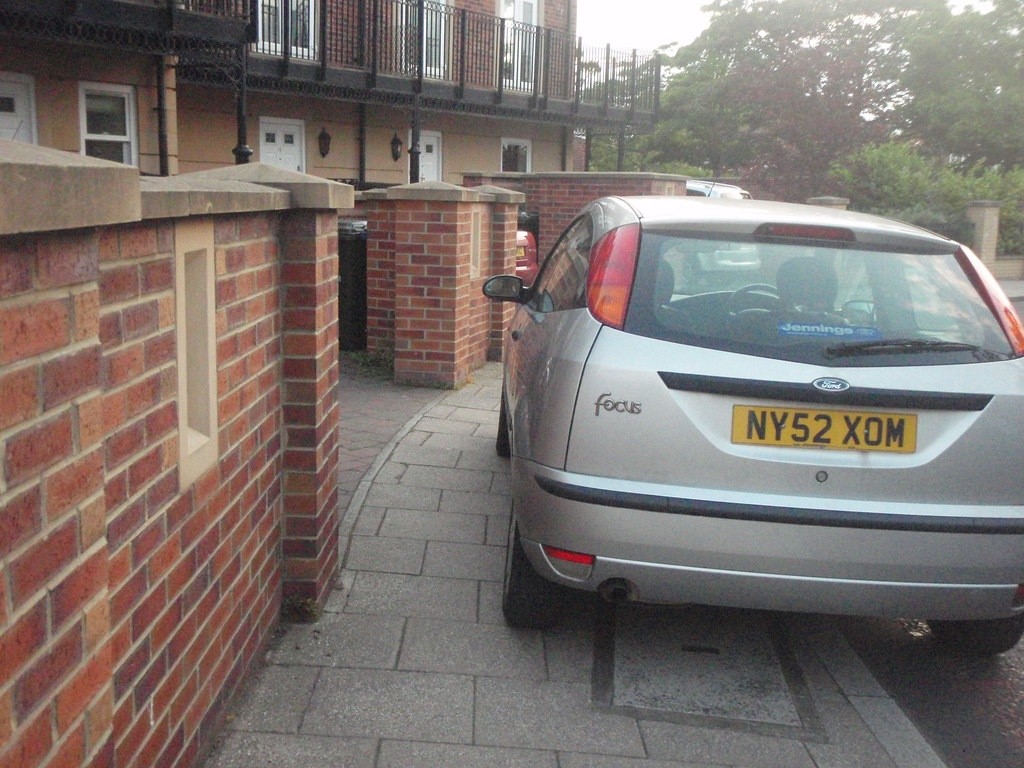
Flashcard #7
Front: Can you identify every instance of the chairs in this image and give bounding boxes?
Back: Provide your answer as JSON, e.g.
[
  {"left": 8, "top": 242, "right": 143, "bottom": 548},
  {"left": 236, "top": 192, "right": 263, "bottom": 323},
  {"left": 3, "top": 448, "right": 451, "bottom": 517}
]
[
  {"left": 653, "top": 257, "right": 696, "bottom": 333},
  {"left": 765, "top": 256, "right": 851, "bottom": 346}
]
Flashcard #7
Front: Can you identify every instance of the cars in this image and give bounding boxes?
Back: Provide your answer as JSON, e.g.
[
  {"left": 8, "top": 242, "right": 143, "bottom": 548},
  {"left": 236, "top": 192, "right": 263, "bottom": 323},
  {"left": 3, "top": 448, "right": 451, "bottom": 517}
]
[{"left": 482, "top": 192, "right": 1024, "bottom": 657}]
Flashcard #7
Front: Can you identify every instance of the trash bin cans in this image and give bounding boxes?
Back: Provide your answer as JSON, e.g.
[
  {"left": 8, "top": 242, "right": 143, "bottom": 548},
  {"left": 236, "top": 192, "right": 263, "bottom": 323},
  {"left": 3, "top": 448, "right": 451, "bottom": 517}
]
[
  {"left": 518, "top": 212, "right": 539, "bottom": 265},
  {"left": 336, "top": 221, "right": 368, "bottom": 351}
]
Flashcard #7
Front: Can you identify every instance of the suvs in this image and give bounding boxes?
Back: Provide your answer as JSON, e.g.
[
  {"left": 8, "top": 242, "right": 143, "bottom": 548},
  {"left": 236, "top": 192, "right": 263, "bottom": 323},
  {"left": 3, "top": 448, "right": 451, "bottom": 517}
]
[{"left": 685, "top": 178, "right": 763, "bottom": 280}]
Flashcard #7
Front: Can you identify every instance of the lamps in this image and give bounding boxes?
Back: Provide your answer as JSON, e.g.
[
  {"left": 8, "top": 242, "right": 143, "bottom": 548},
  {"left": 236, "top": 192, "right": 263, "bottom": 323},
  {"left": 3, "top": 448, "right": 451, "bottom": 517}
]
[
  {"left": 391, "top": 133, "right": 403, "bottom": 161},
  {"left": 318, "top": 127, "right": 332, "bottom": 158}
]
